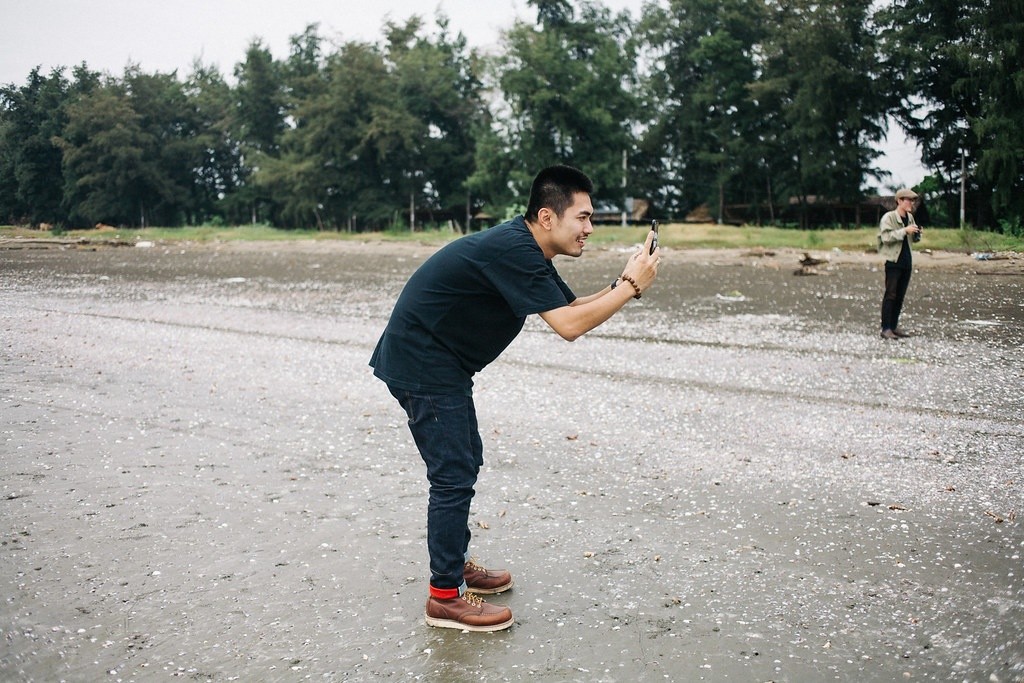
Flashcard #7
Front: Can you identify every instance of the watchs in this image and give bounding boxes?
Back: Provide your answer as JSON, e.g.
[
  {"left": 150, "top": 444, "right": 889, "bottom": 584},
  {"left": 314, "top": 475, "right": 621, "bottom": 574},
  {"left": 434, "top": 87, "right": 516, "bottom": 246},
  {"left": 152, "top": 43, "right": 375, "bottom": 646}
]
[{"left": 611, "top": 278, "right": 618, "bottom": 289}]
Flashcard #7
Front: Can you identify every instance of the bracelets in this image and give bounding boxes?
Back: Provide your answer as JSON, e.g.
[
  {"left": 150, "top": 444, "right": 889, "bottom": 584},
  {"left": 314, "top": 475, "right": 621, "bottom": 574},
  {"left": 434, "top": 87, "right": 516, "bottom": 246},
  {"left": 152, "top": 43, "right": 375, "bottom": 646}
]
[{"left": 621, "top": 274, "right": 641, "bottom": 299}]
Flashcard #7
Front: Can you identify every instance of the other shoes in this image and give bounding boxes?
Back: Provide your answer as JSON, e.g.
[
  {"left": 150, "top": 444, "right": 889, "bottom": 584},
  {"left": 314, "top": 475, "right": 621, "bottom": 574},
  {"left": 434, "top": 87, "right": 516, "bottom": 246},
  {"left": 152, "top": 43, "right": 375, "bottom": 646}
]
[{"left": 880, "top": 329, "right": 909, "bottom": 339}]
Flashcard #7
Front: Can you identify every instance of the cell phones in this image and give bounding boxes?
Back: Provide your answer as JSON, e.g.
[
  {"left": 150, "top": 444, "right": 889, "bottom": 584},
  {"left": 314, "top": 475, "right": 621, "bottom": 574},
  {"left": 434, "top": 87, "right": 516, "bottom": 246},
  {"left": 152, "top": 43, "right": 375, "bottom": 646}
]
[{"left": 650, "top": 218, "right": 658, "bottom": 255}]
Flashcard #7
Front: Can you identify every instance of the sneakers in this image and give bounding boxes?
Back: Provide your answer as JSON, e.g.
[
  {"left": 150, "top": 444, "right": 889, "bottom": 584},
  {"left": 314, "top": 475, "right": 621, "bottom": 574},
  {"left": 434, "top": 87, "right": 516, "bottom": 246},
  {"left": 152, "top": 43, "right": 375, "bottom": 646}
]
[
  {"left": 426, "top": 595, "right": 514, "bottom": 631},
  {"left": 463, "top": 561, "right": 513, "bottom": 593}
]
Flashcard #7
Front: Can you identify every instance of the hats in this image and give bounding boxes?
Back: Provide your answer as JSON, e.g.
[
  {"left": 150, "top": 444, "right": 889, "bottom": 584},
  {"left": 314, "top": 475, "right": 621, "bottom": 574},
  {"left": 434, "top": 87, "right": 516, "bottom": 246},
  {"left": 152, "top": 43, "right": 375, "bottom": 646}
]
[{"left": 894, "top": 188, "right": 918, "bottom": 201}]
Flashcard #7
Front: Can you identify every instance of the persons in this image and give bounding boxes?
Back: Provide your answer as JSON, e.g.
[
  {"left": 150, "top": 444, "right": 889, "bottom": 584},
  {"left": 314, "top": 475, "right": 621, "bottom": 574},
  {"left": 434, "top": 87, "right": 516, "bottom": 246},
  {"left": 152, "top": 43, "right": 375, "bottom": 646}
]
[
  {"left": 880, "top": 189, "right": 922, "bottom": 338},
  {"left": 369, "top": 165, "right": 661, "bottom": 631}
]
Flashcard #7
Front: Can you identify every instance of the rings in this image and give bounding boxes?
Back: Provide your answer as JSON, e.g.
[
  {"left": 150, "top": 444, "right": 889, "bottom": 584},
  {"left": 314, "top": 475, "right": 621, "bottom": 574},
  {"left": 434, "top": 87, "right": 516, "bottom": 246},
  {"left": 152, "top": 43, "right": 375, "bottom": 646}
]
[{"left": 656, "top": 260, "right": 660, "bottom": 266}]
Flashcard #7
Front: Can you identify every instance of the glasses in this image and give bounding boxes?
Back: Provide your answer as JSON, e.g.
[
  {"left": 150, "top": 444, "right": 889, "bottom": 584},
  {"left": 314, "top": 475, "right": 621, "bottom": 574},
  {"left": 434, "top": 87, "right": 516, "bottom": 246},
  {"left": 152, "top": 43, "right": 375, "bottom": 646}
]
[{"left": 905, "top": 197, "right": 916, "bottom": 201}]
